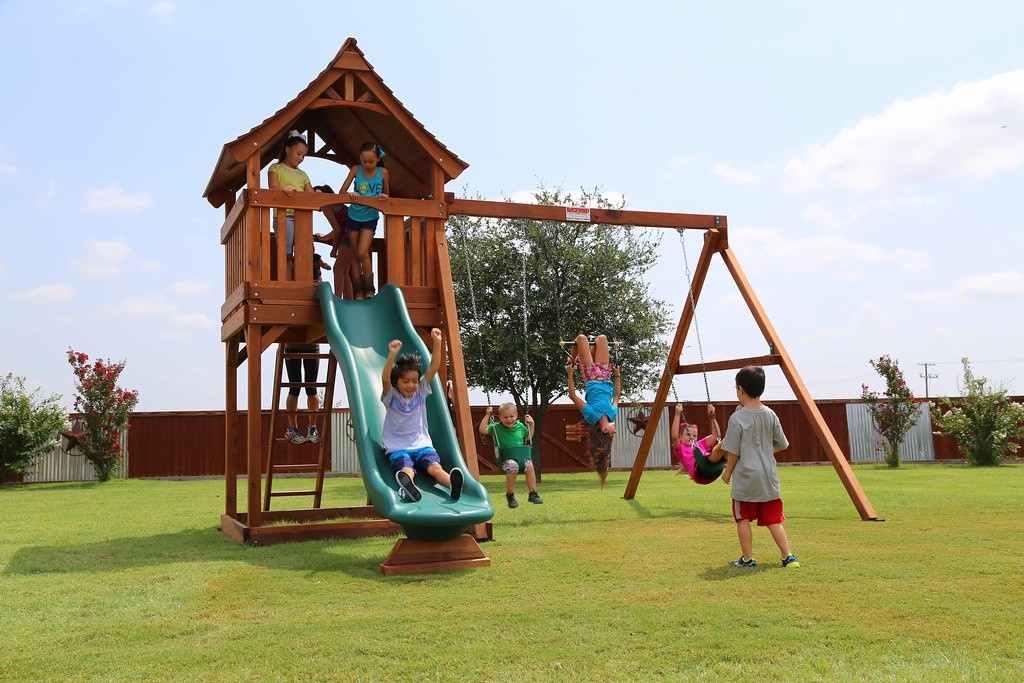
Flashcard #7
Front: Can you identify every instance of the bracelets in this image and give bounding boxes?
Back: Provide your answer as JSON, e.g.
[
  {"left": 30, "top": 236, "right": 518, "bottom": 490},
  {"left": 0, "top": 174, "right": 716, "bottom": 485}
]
[{"left": 614, "top": 375, "right": 620, "bottom": 377}]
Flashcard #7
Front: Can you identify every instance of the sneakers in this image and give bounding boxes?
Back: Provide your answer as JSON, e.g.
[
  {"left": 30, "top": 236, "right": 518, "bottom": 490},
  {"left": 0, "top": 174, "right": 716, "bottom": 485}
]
[
  {"left": 285, "top": 427, "right": 306, "bottom": 444},
  {"left": 781, "top": 553, "right": 800, "bottom": 568},
  {"left": 528, "top": 489, "right": 544, "bottom": 503},
  {"left": 731, "top": 556, "right": 756, "bottom": 567},
  {"left": 506, "top": 492, "right": 518, "bottom": 508},
  {"left": 306, "top": 427, "right": 318, "bottom": 443}
]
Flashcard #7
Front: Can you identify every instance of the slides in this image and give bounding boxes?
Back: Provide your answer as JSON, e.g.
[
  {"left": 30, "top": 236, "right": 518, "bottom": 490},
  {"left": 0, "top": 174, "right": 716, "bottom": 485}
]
[{"left": 316, "top": 280, "right": 495, "bottom": 541}]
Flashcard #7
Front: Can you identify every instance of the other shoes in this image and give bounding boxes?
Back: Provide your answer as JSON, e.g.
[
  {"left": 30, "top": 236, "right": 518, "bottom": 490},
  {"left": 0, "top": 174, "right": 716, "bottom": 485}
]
[
  {"left": 567, "top": 356, "right": 580, "bottom": 365},
  {"left": 396, "top": 470, "right": 422, "bottom": 501},
  {"left": 366, "top": 289, "right": 374, "bottom": 298},
  {"left": 449, "top": 467, "right": 464, "bottom": 500},
  {"left": 355, "top": 293, "right": 364, "bottom": 300}
]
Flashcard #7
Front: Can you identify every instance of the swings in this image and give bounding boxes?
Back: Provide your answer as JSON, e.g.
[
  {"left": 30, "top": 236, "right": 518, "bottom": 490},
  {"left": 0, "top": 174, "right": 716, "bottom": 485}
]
[
  {"left": 625, "top": 223, "right": 727, "bottom": 474},
  {"left": 457, "top": 213, "right": 534, "bottom": 473}
]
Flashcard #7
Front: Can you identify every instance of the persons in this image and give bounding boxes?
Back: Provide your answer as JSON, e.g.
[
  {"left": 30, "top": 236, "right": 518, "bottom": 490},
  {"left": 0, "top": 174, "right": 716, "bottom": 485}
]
[
  {"left": 313, "top": 184, "right": 354, "bottom": 301},
  {"left": 564, "top": 334, "right": 621, "bottom": 489},
  {"left": 380, "top": 328, "right": 464, "bottom": 501},
  {"left": 670, "top": 404, "right": 728, "bottom": 485},
  {"left": 338, "top": 142, "right": 389, "bottom": 301},
  {"left": 479, "top": 402, "right": 543, "bottom": 508},
  {"left": 266, "top": 128, "right": 323, "bottom": 282},
  {"left": 313, "top": 243, "right": 332, "bottom": 287},
  {"left": 283, "top": 343, "right": 320, "bottom": 443},
  {"left": 720, "top": 364, "right": 801, "bottom": 570}
]
